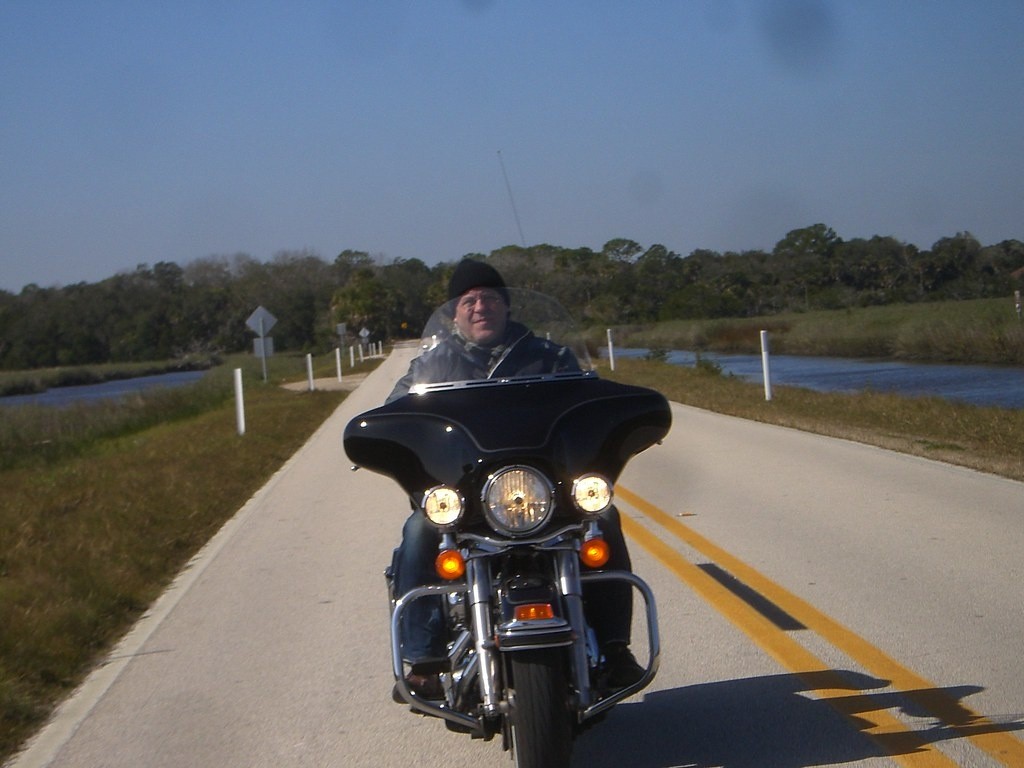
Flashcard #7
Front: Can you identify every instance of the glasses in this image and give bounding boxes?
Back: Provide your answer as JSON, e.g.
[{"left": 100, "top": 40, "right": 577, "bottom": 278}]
[{"left": 459, "top": 293, "right": 502, "bottom": 309}]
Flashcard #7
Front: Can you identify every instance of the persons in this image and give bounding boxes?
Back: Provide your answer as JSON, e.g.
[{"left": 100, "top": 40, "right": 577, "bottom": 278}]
[{"left": 385, "top": 256, "right": 646, "bottom": 704}]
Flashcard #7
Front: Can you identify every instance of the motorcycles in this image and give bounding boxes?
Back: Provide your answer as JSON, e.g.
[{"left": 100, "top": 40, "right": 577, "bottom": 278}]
[{"left": 342, "top": 285, "right": 673, "bottom": 768}]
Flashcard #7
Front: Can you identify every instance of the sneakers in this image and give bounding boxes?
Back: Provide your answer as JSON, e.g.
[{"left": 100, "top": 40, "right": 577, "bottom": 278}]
[
  {"left": 392, "top": 662, "right": 450, "bottom": 704},
  {"left": 590, "top": 643, "right": 645, "bottom": 688}
]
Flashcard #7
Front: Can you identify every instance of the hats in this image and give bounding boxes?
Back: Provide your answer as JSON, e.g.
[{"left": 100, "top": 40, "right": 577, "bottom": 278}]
[{"left": 448, "top": 258, "right": 511, "bottom": 309}]
